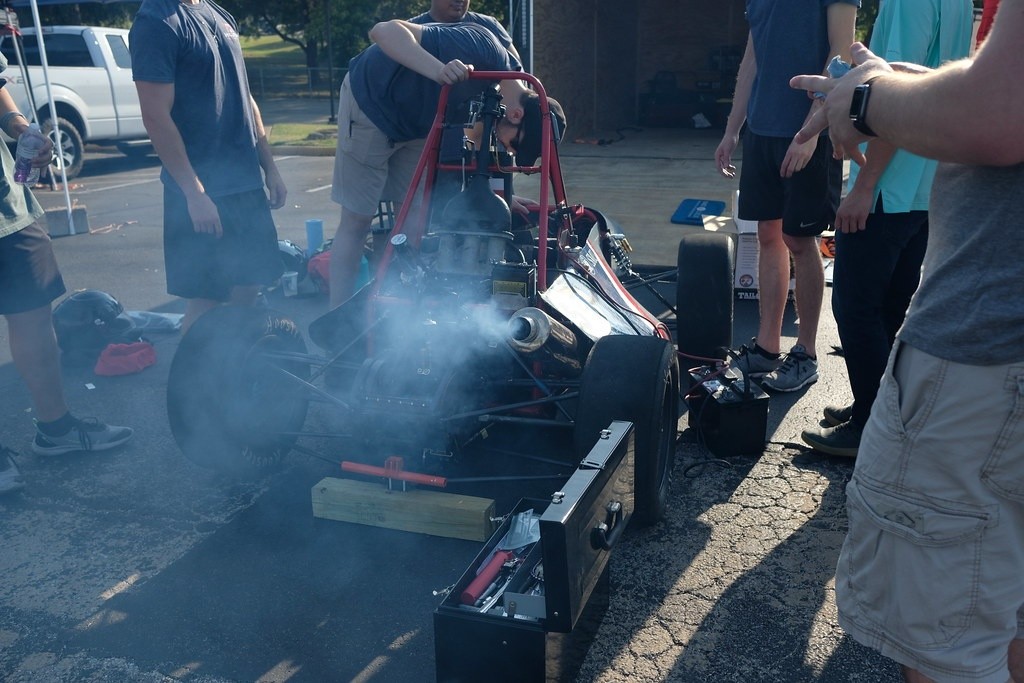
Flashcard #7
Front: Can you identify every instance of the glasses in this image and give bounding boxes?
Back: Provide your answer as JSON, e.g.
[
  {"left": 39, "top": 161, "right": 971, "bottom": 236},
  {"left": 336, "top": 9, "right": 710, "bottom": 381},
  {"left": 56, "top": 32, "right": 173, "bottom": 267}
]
[{"left": 510, "top": 117, "right": 524, "bottom": 150}]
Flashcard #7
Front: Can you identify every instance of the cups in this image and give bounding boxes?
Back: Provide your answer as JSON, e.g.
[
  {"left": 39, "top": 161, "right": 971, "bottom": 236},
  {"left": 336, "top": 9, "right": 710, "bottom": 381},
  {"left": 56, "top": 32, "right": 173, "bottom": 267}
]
[{"left": 282, "top": 271, "right": 299, "bottom": 296}]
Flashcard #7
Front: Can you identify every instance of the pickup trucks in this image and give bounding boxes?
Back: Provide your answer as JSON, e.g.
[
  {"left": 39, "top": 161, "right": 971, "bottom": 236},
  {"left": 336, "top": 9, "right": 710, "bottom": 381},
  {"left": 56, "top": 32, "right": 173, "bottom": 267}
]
[{"left": 0, "top": 25, "right": 162, "bottom": 183}]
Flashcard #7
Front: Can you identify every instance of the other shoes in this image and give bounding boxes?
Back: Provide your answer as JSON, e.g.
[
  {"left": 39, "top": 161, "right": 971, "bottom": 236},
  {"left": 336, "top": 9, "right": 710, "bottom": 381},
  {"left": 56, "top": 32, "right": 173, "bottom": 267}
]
[
  {"left": 824, "top": 405, "right": 856, "bottom": 425},
  {"left": 801, "top": 421, "right": 861, "bottom": 456}
]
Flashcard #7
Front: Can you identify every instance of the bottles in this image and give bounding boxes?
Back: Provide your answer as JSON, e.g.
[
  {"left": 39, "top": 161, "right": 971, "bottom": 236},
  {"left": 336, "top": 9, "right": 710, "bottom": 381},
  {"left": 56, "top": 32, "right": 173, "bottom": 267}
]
[
  {"left": 12, "top": 123, "right": 44, "bottom": 187},
  {"left": 359, "top": 253, "right": 369, "bottom": 289},
  {"left": 305, "top": 218, "right": 326, "bottom": 257}
]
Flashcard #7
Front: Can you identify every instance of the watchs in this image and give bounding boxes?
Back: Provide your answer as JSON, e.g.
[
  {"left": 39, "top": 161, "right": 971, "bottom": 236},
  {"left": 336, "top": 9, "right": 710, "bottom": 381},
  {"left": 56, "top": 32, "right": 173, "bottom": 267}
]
[{"left": 849, "top": 75, "right": 878, "bottom": 138}]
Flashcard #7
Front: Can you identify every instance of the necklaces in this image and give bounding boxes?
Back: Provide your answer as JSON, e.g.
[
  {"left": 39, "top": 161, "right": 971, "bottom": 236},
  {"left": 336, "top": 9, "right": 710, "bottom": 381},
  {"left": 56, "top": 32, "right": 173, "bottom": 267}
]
[{"left": 179, "top": 0, "right": 217, "bottom": 44}]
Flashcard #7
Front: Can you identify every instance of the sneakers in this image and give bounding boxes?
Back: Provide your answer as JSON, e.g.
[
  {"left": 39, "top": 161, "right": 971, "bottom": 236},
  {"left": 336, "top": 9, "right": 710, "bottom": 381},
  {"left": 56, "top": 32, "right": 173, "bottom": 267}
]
[
  {"left": 762, "top": 344, "right": 819, "bottom": 392},
  {"left": 0, "top": 447, "right": 25, "bottom": 494},
  {"left": 724, "top": 339, "right": 788, "bottom": 378},
  {"left": 32, "top": 414, "right": 135, "bottom": 454}
]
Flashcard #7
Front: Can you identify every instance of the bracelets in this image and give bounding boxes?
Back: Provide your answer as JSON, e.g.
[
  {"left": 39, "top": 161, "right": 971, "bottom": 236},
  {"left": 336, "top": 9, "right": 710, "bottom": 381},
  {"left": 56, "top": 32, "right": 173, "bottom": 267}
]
[{"left": 0, "top": 112, "right": 27, "bottom": 138}]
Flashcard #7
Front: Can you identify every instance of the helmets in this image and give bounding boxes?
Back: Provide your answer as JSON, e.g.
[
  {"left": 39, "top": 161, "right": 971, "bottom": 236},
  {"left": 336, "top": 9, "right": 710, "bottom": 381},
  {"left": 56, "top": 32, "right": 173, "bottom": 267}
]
[
  {"left": 278, "top": 240, "right": 308, "bottom": 279},
  {"left": 53, "top": 289, "right": 143, "bottom": 365}
]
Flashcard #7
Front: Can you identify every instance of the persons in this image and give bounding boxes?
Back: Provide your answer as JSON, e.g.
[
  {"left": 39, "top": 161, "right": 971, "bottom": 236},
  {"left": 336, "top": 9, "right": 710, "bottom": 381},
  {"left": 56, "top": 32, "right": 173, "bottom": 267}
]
[
  {"left": 800, "top": 0, "right": 973, "bottom": 457},
  {"left": 128, "top": 0, "right": 287, "bottom": 336},
  {"left": 790, "top": 0, "right": 1024, "bottom": 683},
  {"left": 406, "top": 0, "right": 524, "bottom": 73},
  {"left": 0, "top": 52, "right": 135, "bottom": 493},
  {"left": 714, "top": 0, "right": 859, "bottom": 392},
  {"left": 324, "top": 18, "right": 566, "bottom": 385}
]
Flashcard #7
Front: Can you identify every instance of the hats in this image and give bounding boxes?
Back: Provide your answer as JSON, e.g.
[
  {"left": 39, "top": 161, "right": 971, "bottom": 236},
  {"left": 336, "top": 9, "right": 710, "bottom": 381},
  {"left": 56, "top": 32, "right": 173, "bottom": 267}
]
[{"left": 515, "top": 97, "right": 567, "bottom": 175}]
